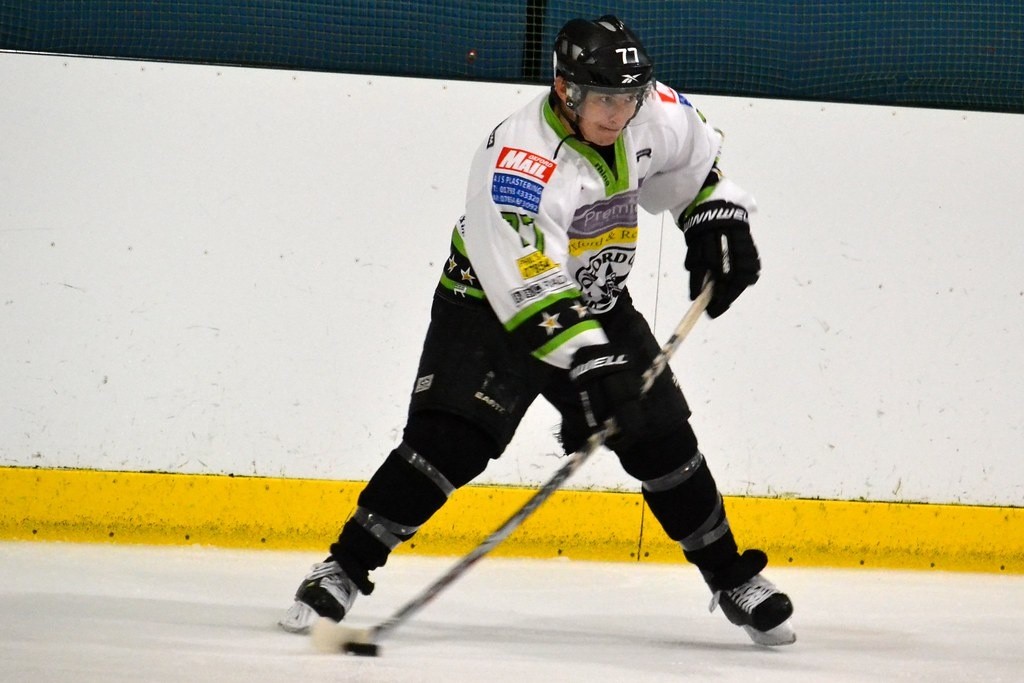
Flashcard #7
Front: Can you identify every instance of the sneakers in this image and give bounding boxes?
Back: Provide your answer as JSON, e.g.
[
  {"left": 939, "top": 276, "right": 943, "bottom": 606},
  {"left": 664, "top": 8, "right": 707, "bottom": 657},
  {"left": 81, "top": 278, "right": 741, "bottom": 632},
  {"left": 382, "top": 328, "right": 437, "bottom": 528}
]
[
  {"left": 710, "top": 548, "right": 797, "bottom": 645},
  {"left": 277, "top": 555, "right": 359, "bottom": 636}
]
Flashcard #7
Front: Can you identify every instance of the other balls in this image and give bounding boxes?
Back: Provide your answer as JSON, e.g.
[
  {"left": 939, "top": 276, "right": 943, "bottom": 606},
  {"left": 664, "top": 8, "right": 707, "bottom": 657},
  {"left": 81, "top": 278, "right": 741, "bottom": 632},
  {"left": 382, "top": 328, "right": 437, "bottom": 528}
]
[{"left": 341, "top": 641, "right": 380, "bottom": 657}]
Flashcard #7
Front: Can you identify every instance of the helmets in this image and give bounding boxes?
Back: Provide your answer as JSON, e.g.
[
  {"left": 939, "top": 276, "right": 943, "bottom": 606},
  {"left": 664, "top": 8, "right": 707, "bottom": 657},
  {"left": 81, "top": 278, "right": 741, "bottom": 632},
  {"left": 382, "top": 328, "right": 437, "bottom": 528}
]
[{"left": 551, "top": 14, "right": 654, "bottom": 109}]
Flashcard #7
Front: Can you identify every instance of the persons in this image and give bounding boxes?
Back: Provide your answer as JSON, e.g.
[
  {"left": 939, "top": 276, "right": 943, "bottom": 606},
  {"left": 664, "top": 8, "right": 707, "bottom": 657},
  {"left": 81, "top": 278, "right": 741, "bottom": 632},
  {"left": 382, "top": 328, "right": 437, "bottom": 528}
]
[{"left": 278, "top": 15, "right": 798, "bottom": 647}]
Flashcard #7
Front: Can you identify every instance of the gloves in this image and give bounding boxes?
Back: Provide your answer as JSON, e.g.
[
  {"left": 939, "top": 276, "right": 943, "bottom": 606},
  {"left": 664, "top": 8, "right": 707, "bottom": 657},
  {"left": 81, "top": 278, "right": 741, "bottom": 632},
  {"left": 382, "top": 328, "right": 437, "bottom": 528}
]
[
  {"left": 560, "top": 341, "right": 649, "bottom": 455},
  {"left": 682, "top": 199, "right": 761, "bottom": 319}
]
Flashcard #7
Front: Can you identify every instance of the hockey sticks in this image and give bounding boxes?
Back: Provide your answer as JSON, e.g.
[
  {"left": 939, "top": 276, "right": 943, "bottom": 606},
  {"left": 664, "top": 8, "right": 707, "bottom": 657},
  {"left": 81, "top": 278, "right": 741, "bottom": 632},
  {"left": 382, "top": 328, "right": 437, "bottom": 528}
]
[{"left": 308, "top": 278, "right": 716, "bottom": 656}]
